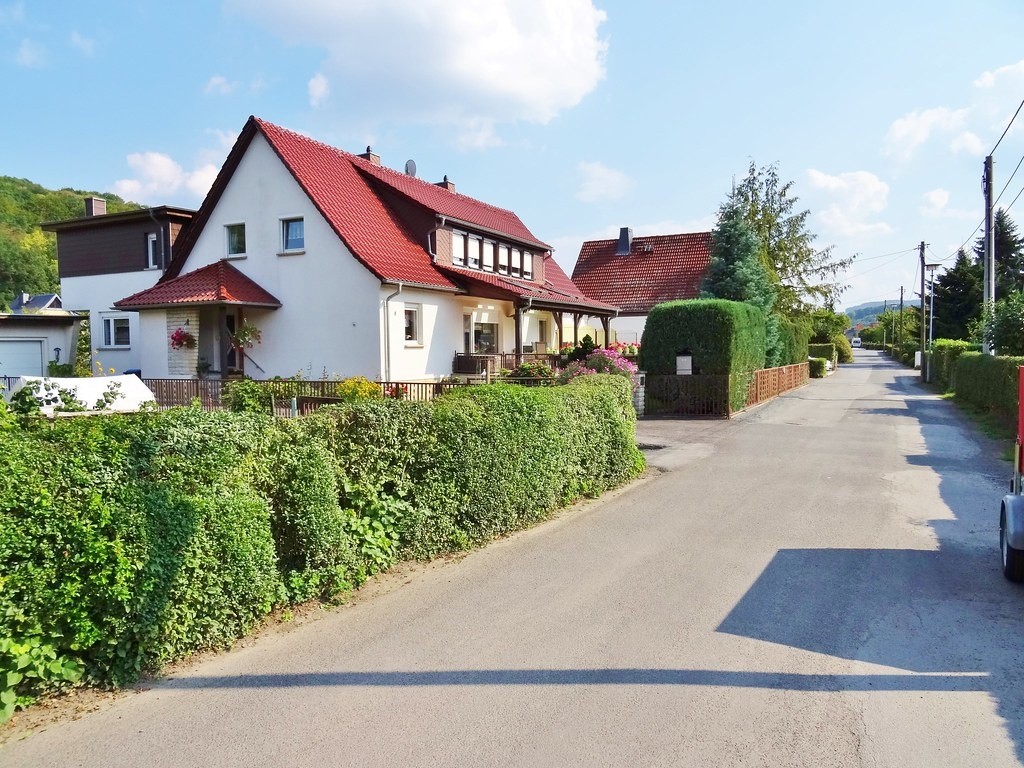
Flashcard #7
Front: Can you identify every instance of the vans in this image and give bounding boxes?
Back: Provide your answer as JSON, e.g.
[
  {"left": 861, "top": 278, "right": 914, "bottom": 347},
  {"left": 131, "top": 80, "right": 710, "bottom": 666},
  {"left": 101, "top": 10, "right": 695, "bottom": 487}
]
[{"left": 851, "top": 337, "right": 862, "bottom": 348}]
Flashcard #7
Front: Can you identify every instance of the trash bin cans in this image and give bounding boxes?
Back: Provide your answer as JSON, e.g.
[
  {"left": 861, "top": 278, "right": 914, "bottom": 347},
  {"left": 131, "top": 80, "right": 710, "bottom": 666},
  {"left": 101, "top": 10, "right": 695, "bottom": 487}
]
[{"left": 123, "top": 368, "right": 141, "bottom": 378}]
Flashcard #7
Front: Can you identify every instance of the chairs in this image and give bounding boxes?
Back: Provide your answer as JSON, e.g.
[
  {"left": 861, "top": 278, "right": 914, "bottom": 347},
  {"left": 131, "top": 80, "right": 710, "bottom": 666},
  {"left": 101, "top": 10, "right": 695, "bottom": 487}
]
[{"left": 535, "top": 342, "right": 550, "bottom": 363}]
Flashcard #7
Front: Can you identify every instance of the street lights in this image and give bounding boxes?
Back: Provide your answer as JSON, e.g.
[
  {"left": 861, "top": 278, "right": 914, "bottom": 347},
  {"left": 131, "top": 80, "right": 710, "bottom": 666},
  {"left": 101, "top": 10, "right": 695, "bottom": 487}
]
[
  {"left": 890, "top": 304, "right": 898, "bottom": 359},
  {"left": 926, "top": 263, "right": 943, "bottom": 354}
]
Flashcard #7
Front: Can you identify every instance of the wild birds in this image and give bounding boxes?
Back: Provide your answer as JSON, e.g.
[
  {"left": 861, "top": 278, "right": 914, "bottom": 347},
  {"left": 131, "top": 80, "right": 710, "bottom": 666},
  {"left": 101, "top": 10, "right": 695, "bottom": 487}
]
[{"left": 481, "top": 369, "right": 487, "bottom": 378}]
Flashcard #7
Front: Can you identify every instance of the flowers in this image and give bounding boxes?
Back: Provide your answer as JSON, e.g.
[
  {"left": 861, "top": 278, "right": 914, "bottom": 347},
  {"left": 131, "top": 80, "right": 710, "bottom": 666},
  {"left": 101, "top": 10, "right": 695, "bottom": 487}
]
[
  {"left": 609, "top": 342, "right": 624, "bottom": 354},
  {"left": 621, "top": 342, "right": 630, "bottom": 356},
  {"left": 629, "top": 343, "right": 640, "bottom": 355},
  {"left": 171, "top": 327, "right": 198, "bottom": 350},
  {"left": 228, "top": 317, "right": 262, "bottom": 353}
]
